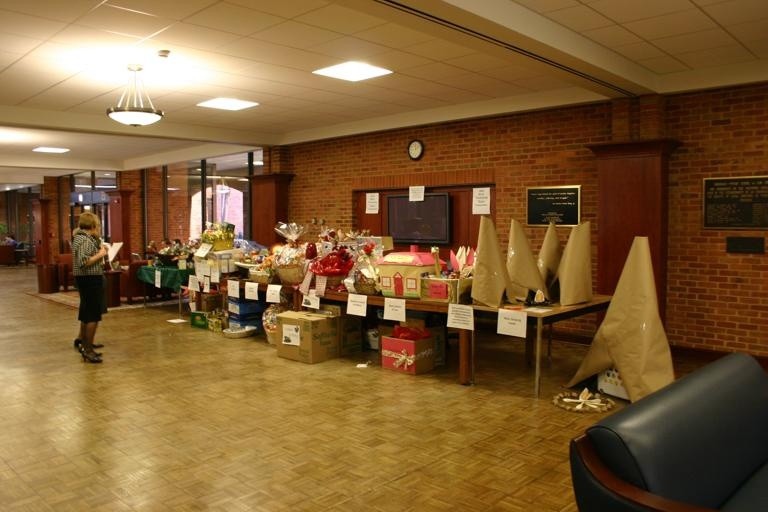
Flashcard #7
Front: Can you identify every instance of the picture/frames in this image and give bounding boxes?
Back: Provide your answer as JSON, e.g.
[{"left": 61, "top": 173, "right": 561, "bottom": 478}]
[
  {"left": 701, "top": 176, "right": 768, "bottom": 232},
  {"left": 525, "top": 184, "right": 581, "bottom": 229}
]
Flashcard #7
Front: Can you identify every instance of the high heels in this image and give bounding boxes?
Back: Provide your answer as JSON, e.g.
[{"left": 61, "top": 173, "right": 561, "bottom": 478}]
[{"left": 74, "top": 337, "right": 105, "bottom": 364}]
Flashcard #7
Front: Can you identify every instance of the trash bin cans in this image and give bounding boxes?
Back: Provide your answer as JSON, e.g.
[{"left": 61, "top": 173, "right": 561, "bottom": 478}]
[
  {"left": 38, "top": 264, "right": 59, "bottom": 293},
  {"left": 100, "top": 271, "right": 122, "bottom": 307}
]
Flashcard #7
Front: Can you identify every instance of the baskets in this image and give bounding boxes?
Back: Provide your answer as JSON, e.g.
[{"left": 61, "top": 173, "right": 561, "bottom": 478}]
[
  {"left": 310, "top": 270, "right": 350, "bottom": 290},
  {"left": 247, "top": 268, "right": 277, "bottom": 285},
  {"left": 274, "top": 265, "right": 308, "bottom": 286}
]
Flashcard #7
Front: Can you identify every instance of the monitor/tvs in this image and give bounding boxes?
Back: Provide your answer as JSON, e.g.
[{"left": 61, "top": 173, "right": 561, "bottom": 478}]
[{"left": 386, "top": 192, "right": 450, "bottom": 244}]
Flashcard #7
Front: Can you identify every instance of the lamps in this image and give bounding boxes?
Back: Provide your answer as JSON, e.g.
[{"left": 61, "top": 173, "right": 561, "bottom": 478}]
[{"left": 106, "top": 64, "right": 164, "bottom": 127}]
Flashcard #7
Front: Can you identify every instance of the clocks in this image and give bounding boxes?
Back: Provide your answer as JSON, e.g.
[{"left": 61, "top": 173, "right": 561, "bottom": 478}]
[{"left": 408, "top": 140, "right": 423, "bottom": 160}]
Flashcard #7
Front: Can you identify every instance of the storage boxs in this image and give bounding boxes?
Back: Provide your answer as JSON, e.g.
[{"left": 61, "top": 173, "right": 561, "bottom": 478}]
[
  {"left": 277, "top": 305, "right": 363, "bottom": 364},
  {"left": 378, "top": 318, "right": 447, "bottom": 375}
]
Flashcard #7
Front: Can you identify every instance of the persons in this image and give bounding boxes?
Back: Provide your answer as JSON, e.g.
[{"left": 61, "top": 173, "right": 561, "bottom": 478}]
[
  {"left": 72, "top": 216, "right": 109, "bottom": 353},
  {"left": 4, "top": 231, "right": 18, "bottom": 247},
  {"left": 72, "top": 212, "right": 103, "bottom": 364},
  {"left": 145, "top": 237, "right": 182, "bottom": 267}
]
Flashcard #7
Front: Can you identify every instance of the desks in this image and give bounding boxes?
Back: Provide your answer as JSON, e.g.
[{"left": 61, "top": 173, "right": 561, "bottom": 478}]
[
  {"left": 221, "top": 279, "right": 298, "bottom": 332},
  {"left": 470, "top": 294, "right": 613, "bottom": 393},
  {"left": 302, "top": 288, "right": 468, "bottom": 386},
  {"left": 138, "top": 266, "right": 196, "bottom": 316}
]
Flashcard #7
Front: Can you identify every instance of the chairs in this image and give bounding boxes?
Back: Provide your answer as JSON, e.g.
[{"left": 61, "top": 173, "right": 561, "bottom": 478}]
[
  {"left": 569, "top": 351, "right": 768, "bottom": 512},
  {"left": 118, "top": 260, "right": 166, "bottom": 304},
  {"left": 55, "top": 253, "right": 74, "bottom": 292}
]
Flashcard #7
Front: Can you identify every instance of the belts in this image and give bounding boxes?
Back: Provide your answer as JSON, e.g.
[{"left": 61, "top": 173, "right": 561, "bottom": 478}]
[{"left": 351, "top": 269, "right": 381, "bottom": 295}]
